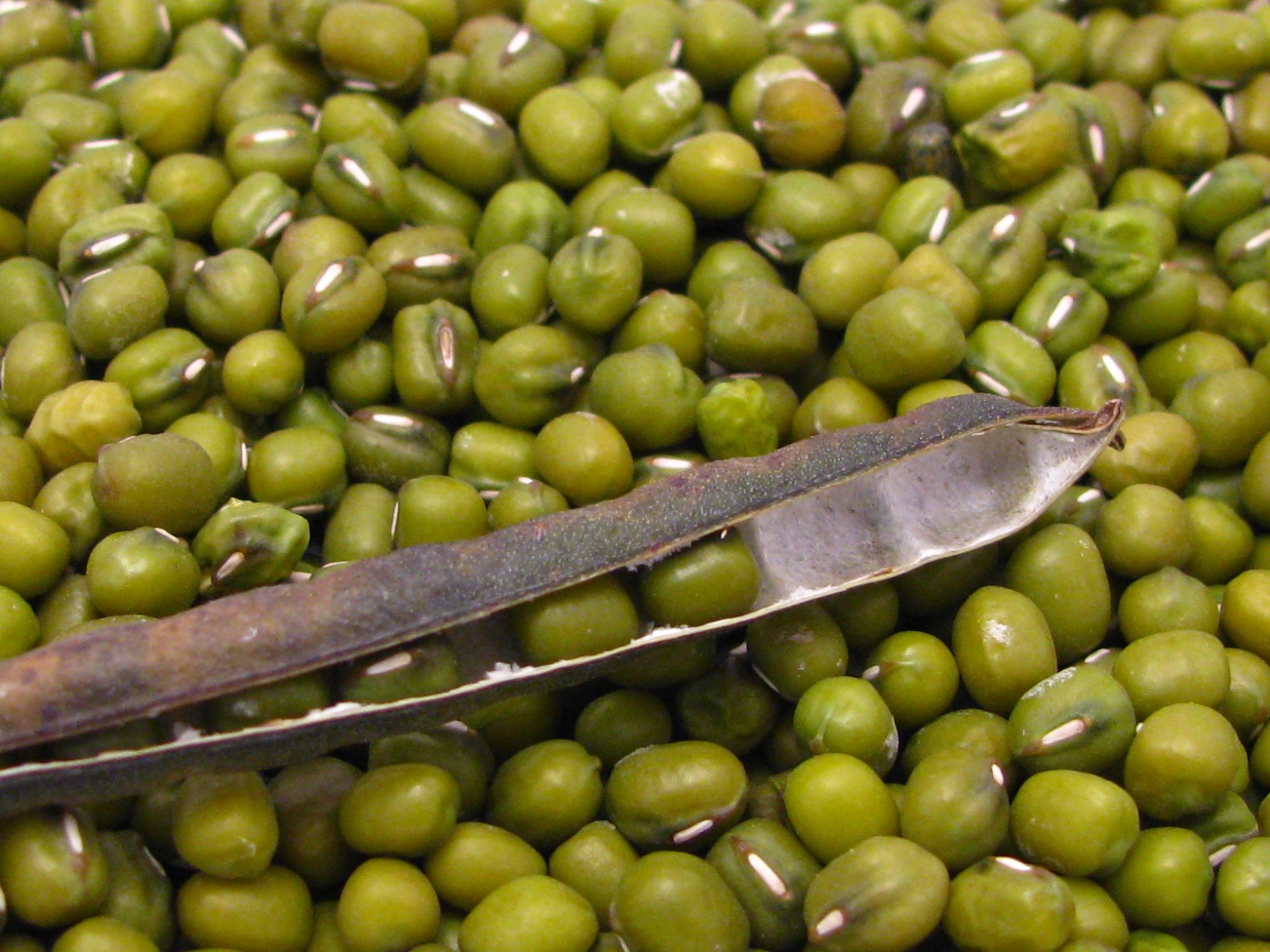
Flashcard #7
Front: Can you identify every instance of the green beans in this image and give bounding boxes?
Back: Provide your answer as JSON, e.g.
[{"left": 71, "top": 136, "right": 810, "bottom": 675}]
[{"left": 0, "top": 0, "right": 1270, "bottom": 952}]
